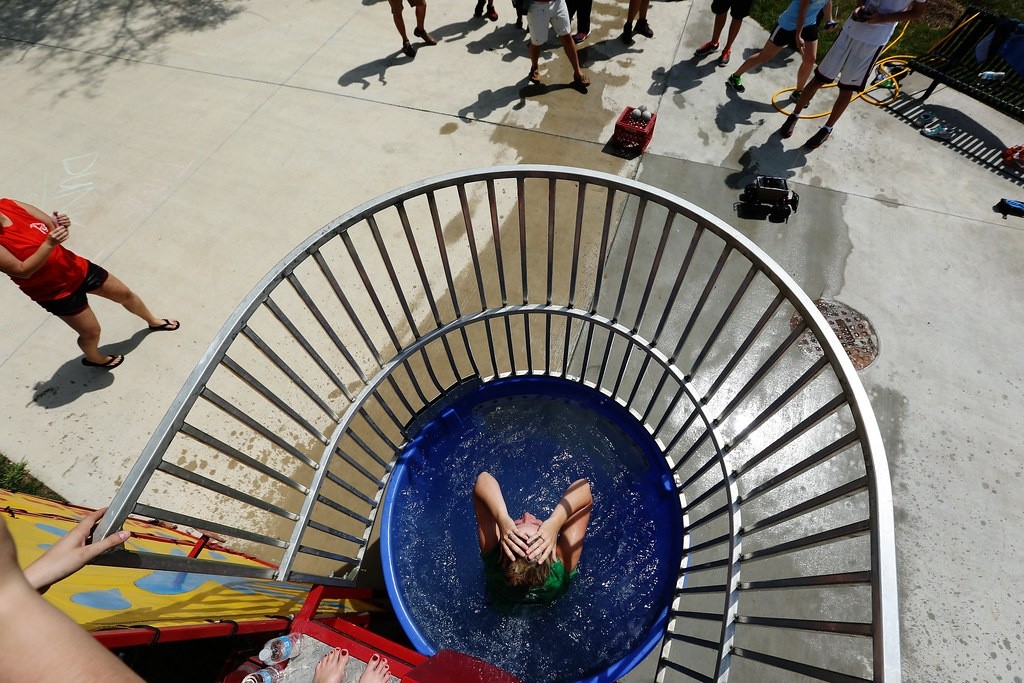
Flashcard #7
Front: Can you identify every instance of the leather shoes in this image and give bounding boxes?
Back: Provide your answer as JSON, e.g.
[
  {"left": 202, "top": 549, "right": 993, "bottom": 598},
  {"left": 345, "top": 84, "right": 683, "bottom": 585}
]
[
  {"left": 402, "top": 41, "right": 414, "bottom": 57},
  {"left": 414, "top": 28, "right": 438, "bottom": 44}
]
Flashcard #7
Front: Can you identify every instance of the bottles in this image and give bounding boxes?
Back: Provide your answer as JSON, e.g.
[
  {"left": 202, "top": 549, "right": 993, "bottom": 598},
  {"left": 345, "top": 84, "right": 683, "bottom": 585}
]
[
  {"left": 242, "top": 664, "right": 285, "bottom": 683},
  {"left": 258, "top": 632, "right": 305, "bottom": 666},
  {"left": 978, "top": 71, "right": 997, "bottom": 80}
]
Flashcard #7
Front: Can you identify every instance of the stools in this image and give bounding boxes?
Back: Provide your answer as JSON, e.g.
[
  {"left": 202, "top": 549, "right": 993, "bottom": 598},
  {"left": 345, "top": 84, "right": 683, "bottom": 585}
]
[{"left": 615, "top": 107, "right": 656, "bottom": 155}]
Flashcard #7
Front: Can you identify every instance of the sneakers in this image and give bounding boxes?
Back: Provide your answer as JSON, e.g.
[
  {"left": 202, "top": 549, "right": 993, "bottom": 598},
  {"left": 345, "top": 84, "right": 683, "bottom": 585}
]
[
  {"left": 695, "top": 40, "right": 719, "bottom": 54},
  {"left": 574, "top": 29, "right": 591, "bottom": 42},
  {"left": 728, "top": 74, "right": 745, "bottom": 92},
  {"left": 790, "top": 91, "right": 810, "bottom": 107},
  {"left": 718, "top": 47, "right": 730, "bottom": 66}
]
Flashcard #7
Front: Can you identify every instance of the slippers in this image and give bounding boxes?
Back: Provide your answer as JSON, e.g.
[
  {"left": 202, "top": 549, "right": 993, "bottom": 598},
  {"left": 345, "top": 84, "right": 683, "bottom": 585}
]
[
  {"left": 149, "top": 319, "right": 180, "bottom": 330},
  {"left": 82, "top": 354, "right": 124, "bottom": 367}
]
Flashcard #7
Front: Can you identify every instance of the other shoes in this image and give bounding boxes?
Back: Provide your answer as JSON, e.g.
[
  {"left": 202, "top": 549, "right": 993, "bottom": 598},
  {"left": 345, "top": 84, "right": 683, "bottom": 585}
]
[
  {"left": 573, "top": 73, "right": 591, "bottom": 84},
  {"left": 806, "top": 127, "right": 833, "bottom": 146},
  {"left": 487, "top": 9, "right": 497, "bottom": 21},
  {"left": 530, "top": 69, "right": 541, "bottom": 81},
  {"left": 517, "top": 19, "right": 522, "bottom": 28},
  {"left": 633, "top": 19, "right": 652, "bottom": 36},
  {"left": 781, "top": 112, "right": 798, "bottom": 138},
  {"left": 623, "top": 22, "right": 634, "bottom": 38},
  {"left": 474, "top": 4, "right": 484, "bottom": 18}
]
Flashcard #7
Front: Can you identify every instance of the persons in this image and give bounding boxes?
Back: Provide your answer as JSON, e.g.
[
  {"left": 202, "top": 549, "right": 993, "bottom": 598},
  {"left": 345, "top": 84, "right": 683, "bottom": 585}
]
[
  {"left": 623, "top": 0, "right": 653, "bottom": 44},
  {"left": 781, "top": 0, "right": 927, "bottom": 149},
  {"left": 0, "top": 507, "right": 391, "bottom": 683},
  {"left": 387, "top": 0, "right": 437, "bottom": 58},
  {"left": 475, "top": 0, "right": 498, "bottom": 21},
  {"left": 0, "top": 198, "right": 180, "bottom": 368},
  {"left": 565, "top": 0, "right": 593, "bottom": 42},
  {"left": 695, "top": 0, "right": 755, "bottom": 66},
  {"left": 474, "top": 472, "right": 593, "bottom": 604},
  {"left": 728, "top": 0, "right": 836, "bottom": 106},
  {"left": 512, "top": 0, "right": 591, "bottom": 88}
]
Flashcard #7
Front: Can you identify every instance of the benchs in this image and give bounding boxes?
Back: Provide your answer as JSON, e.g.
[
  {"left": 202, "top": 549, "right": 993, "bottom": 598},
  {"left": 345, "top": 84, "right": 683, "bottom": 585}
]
[{"left": 907, "top": 7, "right": 1024, "bottom": 124}]
